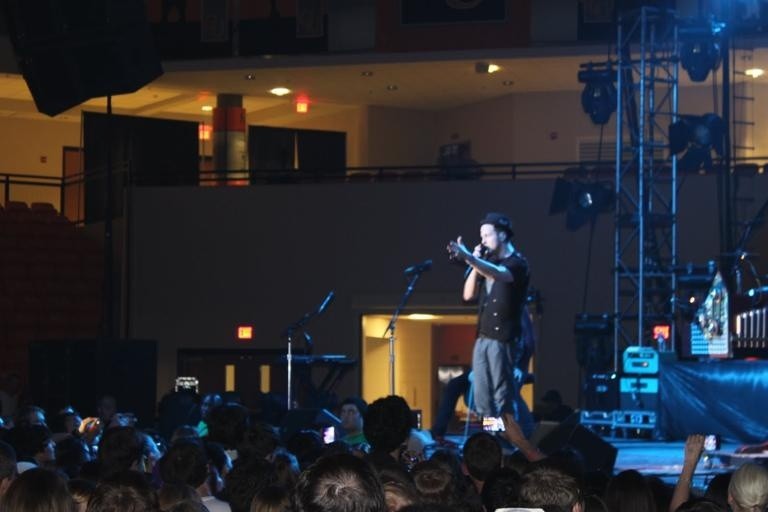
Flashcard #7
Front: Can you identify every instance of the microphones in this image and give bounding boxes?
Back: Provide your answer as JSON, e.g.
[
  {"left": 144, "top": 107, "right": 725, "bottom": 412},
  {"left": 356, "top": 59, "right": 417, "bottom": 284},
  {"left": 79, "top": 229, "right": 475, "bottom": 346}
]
[
  {"left": 404, "top": 259, "right": 432, "bottom": 272},
  {"left": 317, "top": 292, "right": 334, "bottom": 314},
  {"left": 463, "top": 247, "right": 487, "bottom": 282}
]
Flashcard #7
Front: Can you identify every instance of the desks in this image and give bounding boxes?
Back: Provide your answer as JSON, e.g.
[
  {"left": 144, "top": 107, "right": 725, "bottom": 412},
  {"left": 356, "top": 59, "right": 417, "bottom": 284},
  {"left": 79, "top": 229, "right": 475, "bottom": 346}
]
[{"left": 361, "top": 313, "right": 536, "bottom": 431}]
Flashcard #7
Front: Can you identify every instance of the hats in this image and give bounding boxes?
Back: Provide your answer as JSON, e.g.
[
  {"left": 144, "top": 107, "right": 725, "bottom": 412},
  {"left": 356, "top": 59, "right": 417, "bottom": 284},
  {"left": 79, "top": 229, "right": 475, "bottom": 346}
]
[{"left": 479, "top": 210, "right": 516, "bottom": 237}]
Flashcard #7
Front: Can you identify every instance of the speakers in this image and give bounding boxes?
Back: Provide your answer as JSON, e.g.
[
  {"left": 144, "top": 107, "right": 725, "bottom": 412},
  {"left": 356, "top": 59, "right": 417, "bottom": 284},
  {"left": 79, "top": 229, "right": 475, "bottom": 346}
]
[{"left": 508, "top": 420, "right": 618, "bottom": 477}]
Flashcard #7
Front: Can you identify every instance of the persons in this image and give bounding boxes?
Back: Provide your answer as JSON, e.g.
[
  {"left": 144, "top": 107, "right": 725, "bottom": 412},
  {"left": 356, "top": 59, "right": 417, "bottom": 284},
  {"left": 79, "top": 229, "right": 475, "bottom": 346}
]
[
  {"left": 431, "top": 265, "right": 537, "bottom": 445},
  {"left": 446, "top": 212, "right": 532, "bottom": 456},
  {"left": 0, "top": 384, "right": 768, "bottom": 512}
]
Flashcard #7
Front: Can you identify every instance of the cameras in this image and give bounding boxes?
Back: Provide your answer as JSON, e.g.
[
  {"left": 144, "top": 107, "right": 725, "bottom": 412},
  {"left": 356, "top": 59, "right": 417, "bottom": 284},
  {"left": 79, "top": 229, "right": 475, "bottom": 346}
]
[
  {"left": 702, "top": 434, "right": 719, "bottom": 451},
  {"left": 322, "top": 425, "right": 337, "bottom": 445},
  {"left": 482, "top": 415, "right": 507, "bottom": 432}
]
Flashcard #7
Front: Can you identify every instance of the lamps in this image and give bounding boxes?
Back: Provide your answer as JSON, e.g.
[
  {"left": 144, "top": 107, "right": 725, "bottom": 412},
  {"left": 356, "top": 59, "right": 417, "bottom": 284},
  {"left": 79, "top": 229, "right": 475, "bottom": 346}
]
[
  {"left": 679, "top": 12, "right": 730, "bottom": 83},
  {"left": 581, "top": 74, "right": 617, "bottom": 124},
  {"left": 668, "top": 111, "right": 725, "bottom": 174},
  {"left": 545, "top": 167, "right": 615, "bottom": 231}
]
